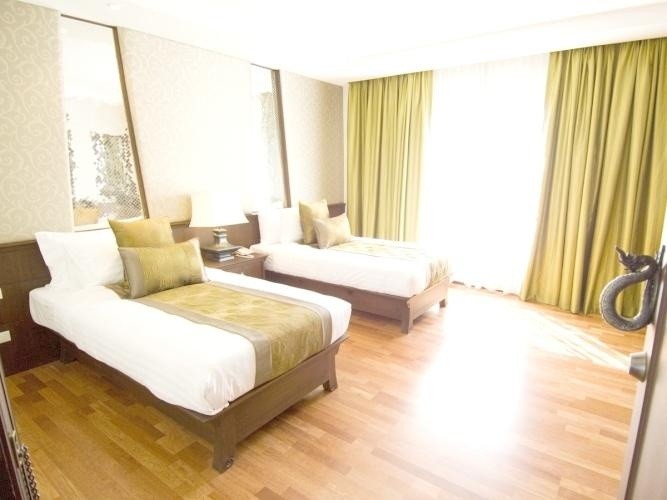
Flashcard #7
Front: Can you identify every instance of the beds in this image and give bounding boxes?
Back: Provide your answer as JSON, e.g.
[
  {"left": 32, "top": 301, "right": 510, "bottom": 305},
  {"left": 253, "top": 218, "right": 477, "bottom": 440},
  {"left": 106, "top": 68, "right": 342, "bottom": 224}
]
[
  {"left": 250, "top": 235, "right": 451, "bottom": 337},
  {"left": 27, "top": 265, "right": 353, "bottom": 475}
]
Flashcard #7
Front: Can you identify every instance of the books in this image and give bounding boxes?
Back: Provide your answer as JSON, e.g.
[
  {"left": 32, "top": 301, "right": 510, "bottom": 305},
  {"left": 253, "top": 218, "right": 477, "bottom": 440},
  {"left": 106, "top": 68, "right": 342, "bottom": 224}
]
[
  {"left": 207, "top": 256, "right": 234, "bottom": 262},
  {"left": 210, "top": 253, "right": 231, "bottom": 257}
]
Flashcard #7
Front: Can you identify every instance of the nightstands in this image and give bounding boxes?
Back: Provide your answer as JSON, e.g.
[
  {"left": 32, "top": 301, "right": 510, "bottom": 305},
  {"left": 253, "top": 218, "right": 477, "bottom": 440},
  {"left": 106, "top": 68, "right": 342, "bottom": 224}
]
[{"left": 214, "top": 251, "right": 272, "bottom": 283}]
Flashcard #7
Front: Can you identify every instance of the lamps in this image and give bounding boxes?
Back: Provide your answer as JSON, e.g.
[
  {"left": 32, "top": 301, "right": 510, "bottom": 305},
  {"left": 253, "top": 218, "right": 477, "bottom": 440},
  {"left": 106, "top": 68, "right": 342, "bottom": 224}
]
[{"left": 188, "top": 190, "right": 253, "bottom": 264}]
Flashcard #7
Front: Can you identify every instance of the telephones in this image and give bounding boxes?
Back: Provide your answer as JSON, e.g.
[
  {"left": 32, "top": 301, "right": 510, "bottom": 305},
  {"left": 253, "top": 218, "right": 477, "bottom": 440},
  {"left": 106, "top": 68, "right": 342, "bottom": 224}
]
[{"left": 231, "top": 246, "right": 253, "bottom": 256}]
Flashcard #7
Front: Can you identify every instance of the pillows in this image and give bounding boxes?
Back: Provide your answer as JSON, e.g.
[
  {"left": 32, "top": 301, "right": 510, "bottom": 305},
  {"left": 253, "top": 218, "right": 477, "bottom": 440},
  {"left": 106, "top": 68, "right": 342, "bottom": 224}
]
[
  {"left": 113, "top": 235, "right": 210, "bottom": 300},
  {"left": 310, "top": 208, "right": 354, "bottom": 247},
  {"left": 106, "top": 213, "right": 176, "bottom": 291},
  {"left": 256, "top": 205, "right": 304, "bottom": 247},
  {"left": 292, "top": 197, "right": 330, "bottom": 245},
  {"left": 30, "top": 228, "right": 123, "bottom": 288}
]
